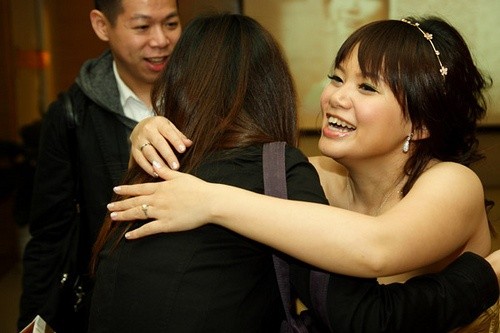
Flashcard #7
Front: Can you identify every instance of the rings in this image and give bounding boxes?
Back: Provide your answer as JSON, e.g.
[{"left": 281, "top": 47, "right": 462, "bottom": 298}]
[
  {"left": 142, "top": 203, "right": 150, "bottom": 219},
  {"left": 138, "top": 142, "right": 150, "bottom": 151}
]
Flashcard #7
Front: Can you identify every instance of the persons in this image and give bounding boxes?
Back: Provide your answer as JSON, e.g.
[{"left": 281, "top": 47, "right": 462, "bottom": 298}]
[
  {"left": 88, "top": 14, "right": 500, "bottom": 333},
  {"left": 10, "top": 0, "right": 187, "bottom": 331},
  {"left": 107, "top": 10, "right": 500, "bottom": 333}
]
